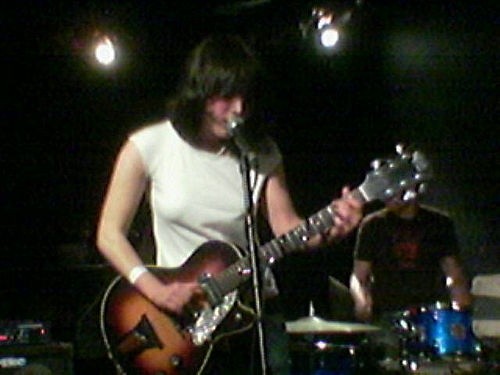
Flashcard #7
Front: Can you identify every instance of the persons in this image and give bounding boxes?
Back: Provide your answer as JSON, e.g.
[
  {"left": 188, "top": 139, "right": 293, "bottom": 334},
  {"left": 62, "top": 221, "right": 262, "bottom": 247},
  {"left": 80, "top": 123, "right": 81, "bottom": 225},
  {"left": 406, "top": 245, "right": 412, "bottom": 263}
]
[
  {"left": 94, "top": 33, "right": 364, "bottom": 375},
  {"left": 350, "top": 183, "right": 471, "bottom": 320}
]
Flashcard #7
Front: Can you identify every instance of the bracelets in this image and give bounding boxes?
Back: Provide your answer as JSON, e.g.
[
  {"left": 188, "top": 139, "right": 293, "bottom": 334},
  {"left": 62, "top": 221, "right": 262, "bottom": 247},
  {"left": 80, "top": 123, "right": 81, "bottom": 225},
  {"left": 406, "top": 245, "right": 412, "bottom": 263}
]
[{"left": 127, "top": 265, "right": 147, "bottom": 284}]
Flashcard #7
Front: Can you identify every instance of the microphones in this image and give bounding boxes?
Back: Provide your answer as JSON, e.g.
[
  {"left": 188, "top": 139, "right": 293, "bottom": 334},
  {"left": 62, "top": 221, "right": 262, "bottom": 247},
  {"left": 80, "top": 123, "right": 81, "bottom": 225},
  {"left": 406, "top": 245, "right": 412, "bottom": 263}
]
[{"left": 227, "top": 117, "right": 259, "bottom": 168}]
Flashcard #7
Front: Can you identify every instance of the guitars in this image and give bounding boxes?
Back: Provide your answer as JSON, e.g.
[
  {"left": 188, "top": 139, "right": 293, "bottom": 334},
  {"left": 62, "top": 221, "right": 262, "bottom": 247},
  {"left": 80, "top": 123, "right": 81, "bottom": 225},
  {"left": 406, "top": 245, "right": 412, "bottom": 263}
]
[{"left": 98, "top": 142, "right": 435, "bottom": 375}]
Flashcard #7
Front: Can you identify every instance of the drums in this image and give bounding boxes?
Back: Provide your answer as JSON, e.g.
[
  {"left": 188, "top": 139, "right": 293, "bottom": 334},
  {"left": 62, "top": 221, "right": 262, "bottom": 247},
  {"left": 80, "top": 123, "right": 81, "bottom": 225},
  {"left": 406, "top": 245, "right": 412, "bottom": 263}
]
[
  {"left": 290, "top": 342, "right": 357, "bottom": 375},
  {"left": 391, "top": 301, "right": 483, "bottom": 375}
]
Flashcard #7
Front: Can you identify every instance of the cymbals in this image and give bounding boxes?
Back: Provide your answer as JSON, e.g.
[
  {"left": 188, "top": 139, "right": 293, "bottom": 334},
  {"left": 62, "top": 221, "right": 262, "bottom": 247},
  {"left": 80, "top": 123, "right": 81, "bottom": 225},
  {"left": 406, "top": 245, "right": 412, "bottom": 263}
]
[{"left": 285, "top": 316, "right": 382, "bottom": 334}]
